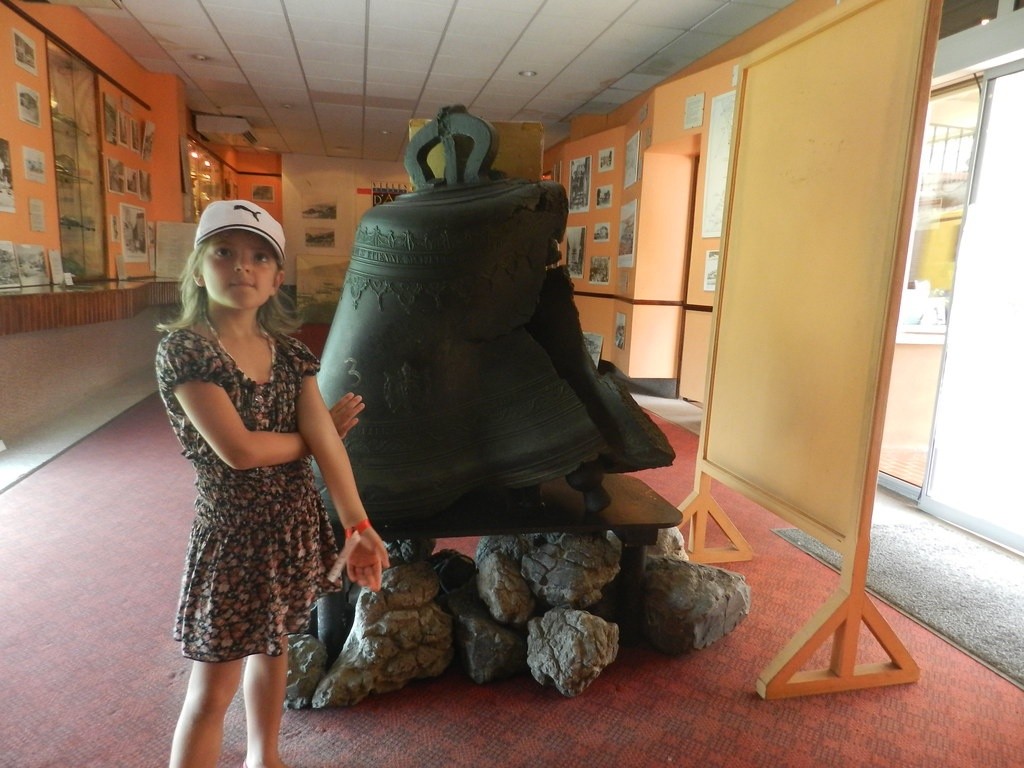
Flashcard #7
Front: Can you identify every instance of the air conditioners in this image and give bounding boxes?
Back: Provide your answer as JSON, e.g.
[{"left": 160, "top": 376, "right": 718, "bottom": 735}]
[{"left": 196, "top": 114, "right": 258, "bottom": 148}]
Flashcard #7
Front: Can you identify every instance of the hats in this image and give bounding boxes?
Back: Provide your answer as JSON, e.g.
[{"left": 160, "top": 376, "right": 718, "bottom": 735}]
[{"left": 197, "top": 199, "right": 287, "bottom": 265}]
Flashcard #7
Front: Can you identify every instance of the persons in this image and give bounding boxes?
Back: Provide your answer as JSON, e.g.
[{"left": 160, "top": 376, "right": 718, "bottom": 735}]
[{"left": 154, "top": 197, "right": 391, "bottom": 768}]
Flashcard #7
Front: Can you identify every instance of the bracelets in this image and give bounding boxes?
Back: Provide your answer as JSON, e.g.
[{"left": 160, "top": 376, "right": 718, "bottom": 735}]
[{"left": 344, "top": 518, "right": 372, "bottom": 538}]
